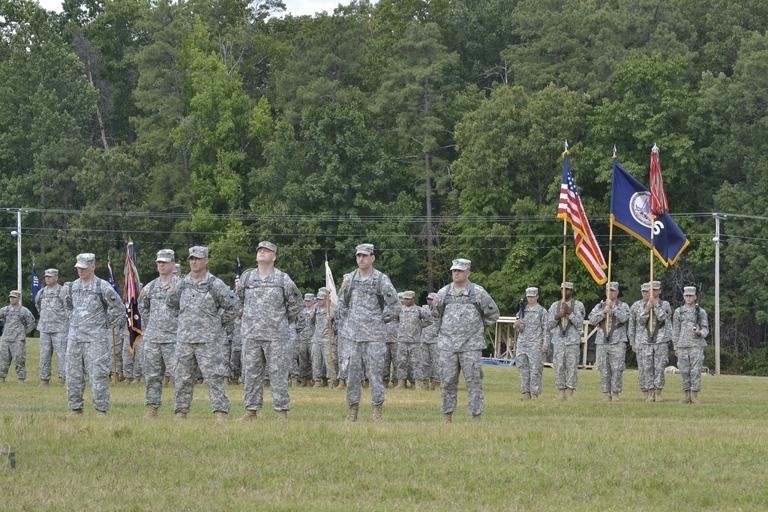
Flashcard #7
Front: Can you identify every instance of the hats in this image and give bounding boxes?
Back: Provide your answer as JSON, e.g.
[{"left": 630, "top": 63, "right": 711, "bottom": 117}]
[
  {"left": 642, "top": 283, "right": 650, "bottom": 291},
  {"left": 684, "top": 286, "right": 697, "bottom": 296},
  {"left": 189, "top": 246, "right": 209, "bottom": 259},
  {"left": 606, "top": 282, "right": 619, "bottom": 291},
  {"left": 8, "top": 290, "right": 20, "bottom": 298},
  {"left": 43, "top": 268, "right": 58, "bottom": 277},
  {"left": 426, "top": 293, "right": 437, "bottom": 300},
  {"left": 403, "top": 290, "right": 415, "bottom": 300},
  {"left": 317, "top": 291, "right": 326, "bottom": 299},
  {"left": 155, "top": 249, "right": 175, "bottom": 263},
  {"left": 450, "top": 259, "right": 471, "bottom": 271},
  {"left": 319, "top": 287, "right": 331, "bottom": 294},
  {"left": 561, "top": 283, "right": 573, "bottom": 290},
  {"left": 173, "top": 264, "right": 181, "bottom": 275},
  {"left": 305, "top": 293, "right": 315, "bottom": 301},
  {"left": 256, "top": 241, "right": 277, "bottom": 253},
  {"left": 650, "top": 280, "right": 661, "bottom": 290},
  {"left": 73, "top": 253, "right": 95, "bottom": 269},
  {"left": 526, "top": 287, "right": 539, "bottom": 297},
  {"left": 398, "top": 292, "right": 403, "bottom": 300},
  {"left": 355, "top": 244, "right": 374, "bottom": 256}
]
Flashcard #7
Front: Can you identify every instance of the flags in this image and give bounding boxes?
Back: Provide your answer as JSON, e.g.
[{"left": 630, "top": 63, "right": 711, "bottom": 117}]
[
  {"left": 325, "top": 260, "right": 337, "bottom": 319},
  {"left": 105, "top": 260, "right": 120, "bottom": 300},
  {"left": 123, "top": 240, "right": 143, "bottom": 353},
  {"left": 608, "top": 157, "right": 690, "bottom": 267},
  {"left": 558, "top": 151, "right": 608, "bottom": 285},
  {"left": 235, "top": 261, "right": 243, "bottom": 279},
  {"left": 649, "top": 142, "right": 668, "bottom": 221},
  {"left": 31, "top": 263, "right": 42, "bottom": 303}
]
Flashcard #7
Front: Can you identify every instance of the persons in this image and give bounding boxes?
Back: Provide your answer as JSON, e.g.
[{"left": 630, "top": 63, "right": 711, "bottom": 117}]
[
  {"left": 311, "top": 293, "right": 337, "bottom": 390},
  {"left": 421, "top": 292, "right": 442, "bottom": 391},
  {"left": 398, "top": 293, "right": 404, "bottom": 306},
  {"left": 298, "top": 294, "right": 317, "bottom": 387},
  {"left": 106, "top": 284, "right": 144, "bottom": 384},
  {"left": 0, "top": 291, "right": 35, "bottom": 383},
  {"left": 318, "top": 288, "right": 332, "bottom": 295},
  {"left": 138, "top": 249, "right": 184, "bottom": 419},
  {"left": 229, "top": 313, "right": 243, "bottom": 385},
  {"left": 514, "top": 287, "right": 550, "bottom": 400},
  {"left": 628, "top": 283, "right": 650, "bottom": 391},
  {"left": 35, "top": 269, "right": 67, "bottom": 386},
  {"left": 673, "top": 286, "right": 708, "bottom": 404},
  {"left": 398, "top": 291, "right": 433, "bottom": 390},
  {"left": 588, "top": 282, "right": 630, "bottom": 400},
  {"left": 62, "top": 254, "right": 126, "bottom": 418},
  {"left": 166, "top": 246, "right": 242, "bottom": 420},
  {"left": 174, "top": 264, "right": 181, "bottom": 279},
  {"left": 336, "top": 243, "right": 403, "bottom": 424},
  {"left": 383, "top": 319, "right": 399, "bottom": 389},
  {"left": 548, "top": 282, "right": 586, "bottom": 400},
  {"left": 643, "top": 280, "right": 673, "bottom": 402},
  {"left": 428, "top": 258, "right": 500, "bottom": 427},
  {"left": 235, "top": 242, "right": 305, "bottom": 424}
]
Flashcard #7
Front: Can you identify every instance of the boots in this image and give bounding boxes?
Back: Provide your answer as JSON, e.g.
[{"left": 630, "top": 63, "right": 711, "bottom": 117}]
[
  {"left": 555, "top": 389, "right": 565, "bottom": 401},
  {"left": 612, "top": 392, "right": 622, "bottom": 402},
  {"left": 264, "top": 379, "right": 271, "bottom": 386},
  {"left": 344, "top": 404, "right": 358, "bottom": 423},
  {"left": 67, "top": 408, "right": 84, "bottom": 416},
  {"left": 440, "top": 412, "right": 452, "bottom": 424},
  {"left": 0, "top": 377, "right": 6, "bottom": 383},
  {"left": 680, "top": 389, "right": 691, "bottom": 403},
  {"left": 471, "top": 414, "right": 482, "bottom": 424},
  {"left": 288, "top": 376, "right": 348, "bottom": 389},
  {"left": 519, "top": 391, "right": 531, "bottom": 401},
  {"left": 193, "top": 377, "right": 246, "bottom": 387},
  {"left": 362, "top": 378, "right": 442, "bottom": 390},
  {"left": 175, "top": 409, "right": 188, "bottom": 418},
  {"left": 110, "top": 373, "right": 146, "bottom": 384},
  {"left": 18, "top": 378, "right": 25, "bottom": 384},
  {"left": 214, "top": 412, "right": 227, "bottom": 422},
  {"left": 655, "top": 388, "right": 664, "bottom": 402},
  {"left": 531, "top": 393, "right": 539, "bottom": 402},
  {"left": 57, "top": 377, "right": 65, "bottom": 385},
  {"left": 96, "top": 410, "right": 106, "bottom": 418},
  {"left": 162, "top": 376, "right": 175, "bottom": 384},
  {"left": 278, "top": 410, "right": 288, "bottom": 420},
  {"left": 644, "top": 390, "right": 655, "bottom": 402},
  {"left": 234, "top": 409, "right": 257, "bottom": 422},
  {"left": 38, "top": 379, "right": 49, "bottom": 387},
  {"left": 371, "top": 404, "right": 382, "bottom": 423},
  {"left": 601, "top": 393, "right": 612, "bottom": 402},
  {"left": 691, "top": 391, "right": 701, "bottom": 405},
  {"left": 565, "top": 389, "right": 574, "bottom": 401},
  {"left": 141, "top": 407, "right": 158, "bottom": 417}
]
